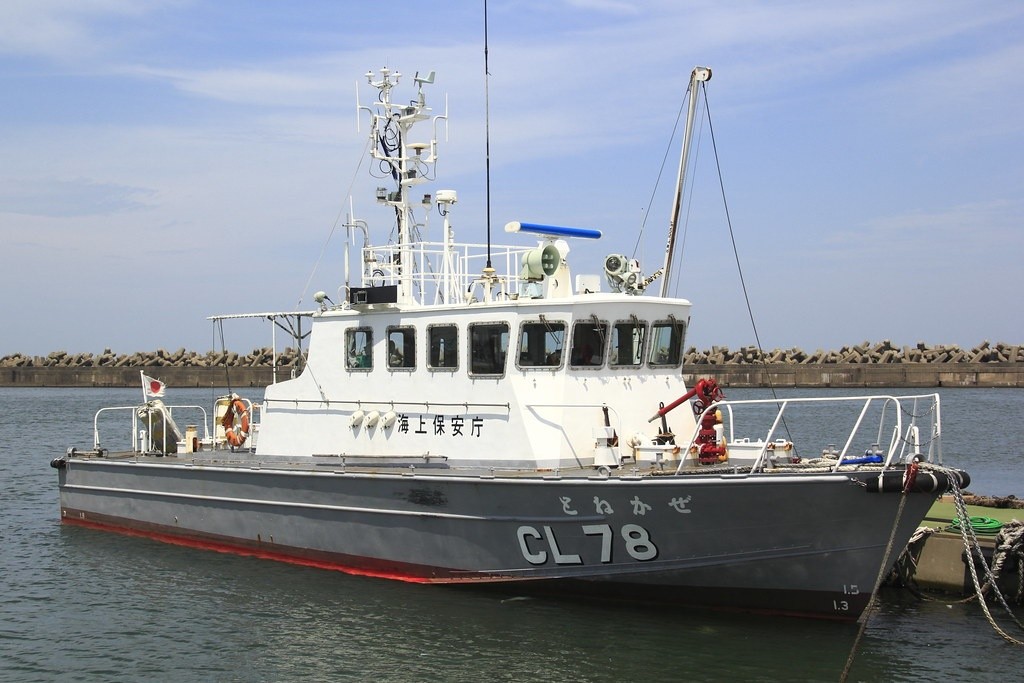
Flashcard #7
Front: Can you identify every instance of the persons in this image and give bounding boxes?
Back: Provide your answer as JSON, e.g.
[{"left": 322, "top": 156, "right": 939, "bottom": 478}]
[
  {"left": 348, "top": 347, "right": 371, "bottom": 368},
  {"left": 389, "top": 341, "right": 402, "bottom": 367},
  {"left": 572, "top": 344, "right": 595, "bottom": 365}
]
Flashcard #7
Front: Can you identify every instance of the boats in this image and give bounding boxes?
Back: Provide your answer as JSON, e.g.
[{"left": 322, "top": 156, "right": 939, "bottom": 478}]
[{"left": 47, "top": 0, "right": 973, "bottom": 615}]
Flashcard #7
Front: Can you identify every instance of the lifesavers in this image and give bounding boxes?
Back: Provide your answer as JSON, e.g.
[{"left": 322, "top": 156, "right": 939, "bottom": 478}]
[{"left": 224, "top": 399, "right": 249, "bottom": 447}]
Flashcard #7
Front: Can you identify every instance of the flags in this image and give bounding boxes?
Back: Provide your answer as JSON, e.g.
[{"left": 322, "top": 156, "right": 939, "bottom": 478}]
[{"left": 144, "top": 375, "right": 165, "bottom": 397}]
